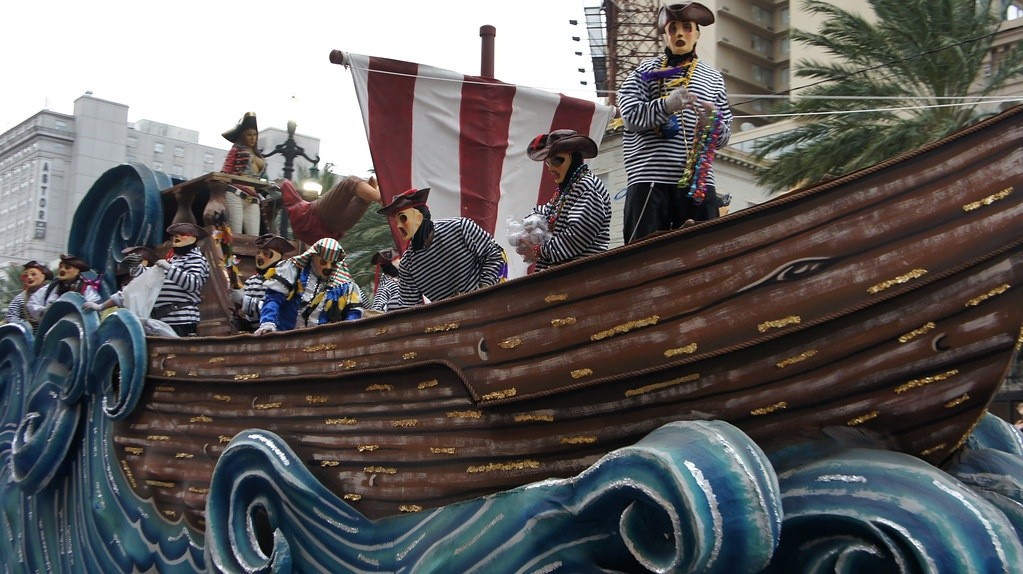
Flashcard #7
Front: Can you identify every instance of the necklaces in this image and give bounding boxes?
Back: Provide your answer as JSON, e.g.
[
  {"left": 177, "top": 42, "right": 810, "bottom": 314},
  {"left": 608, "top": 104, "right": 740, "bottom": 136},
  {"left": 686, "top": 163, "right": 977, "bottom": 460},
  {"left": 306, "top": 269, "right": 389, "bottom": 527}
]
[
  {"left": 656, "top": 53, "right": 699, "bottom": 102},
  {"left": 676, "top": 104, "right": 722, "bottom": 206},
  {"left": 541, "top": 162, "right": 588, "bottom": 232}
]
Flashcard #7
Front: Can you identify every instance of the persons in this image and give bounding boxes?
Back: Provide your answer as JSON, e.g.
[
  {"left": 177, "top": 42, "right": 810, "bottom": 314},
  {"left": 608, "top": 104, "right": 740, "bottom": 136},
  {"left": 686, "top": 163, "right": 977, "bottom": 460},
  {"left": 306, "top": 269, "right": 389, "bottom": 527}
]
[
  {"left": 227, "top": 230, "right": 293, "bottom": 331},
  {"left": 220, "top": 110, "right": 267, "bottom": 239},
  {"left": 619, "top": 2, "right": 735, "bottom": 239},
  {"left": 83, "top": 246, "right": 157, "bottom": 317},
  {"left": 7, "top": 261, "right": 54, "bottom": 337},
  {"left": 146, "top": 222, "right": 213, "bottom": 339},
  {"left": 388, "top": 187, "right": 504, "bottom": 305},
  {"left": 255, "top": 237, "right": 362, "bottom": 337},
  {"left": 516, "top": 129, "right": 613, "bottom": 274},
  {"left": 26, "top": 250, "right": 99, "bottom": 323},
  {"left": 371, "top": 247, "right": 404, "bottom": 313}
]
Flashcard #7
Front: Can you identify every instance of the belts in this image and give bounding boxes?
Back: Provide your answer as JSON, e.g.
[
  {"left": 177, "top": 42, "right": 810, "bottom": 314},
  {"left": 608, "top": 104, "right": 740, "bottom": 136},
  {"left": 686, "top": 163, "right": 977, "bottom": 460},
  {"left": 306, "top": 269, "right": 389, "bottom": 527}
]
[{"left": 225, "top": 186, "right": 258, "bottom": 203}]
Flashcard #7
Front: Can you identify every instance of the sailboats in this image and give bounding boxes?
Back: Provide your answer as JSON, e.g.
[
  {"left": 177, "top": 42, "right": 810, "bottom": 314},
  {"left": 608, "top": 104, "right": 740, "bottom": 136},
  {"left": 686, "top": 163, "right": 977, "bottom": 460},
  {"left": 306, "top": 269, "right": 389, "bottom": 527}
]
[{"left": 0, "top": 0, "right": 1023, "bottom": 524}]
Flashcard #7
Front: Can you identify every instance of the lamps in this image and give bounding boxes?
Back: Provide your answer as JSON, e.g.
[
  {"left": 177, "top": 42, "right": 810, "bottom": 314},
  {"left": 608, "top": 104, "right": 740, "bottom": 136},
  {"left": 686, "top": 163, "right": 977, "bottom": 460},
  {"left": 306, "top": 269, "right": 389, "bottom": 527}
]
[
  {"left": 580, "top": 81, "right": 587, "bottom": 85},
  {"left": 575, "top": 52, "right": 582, "bottom": 56},
  {"left": 569, "top": 19, "right": 578, "bottom": 25},
  {"left": 572, "top": 36, "right": 579, "bottom": 41},
  {"left": 578, "top": 69, "right": 585, "bottom": 73}
]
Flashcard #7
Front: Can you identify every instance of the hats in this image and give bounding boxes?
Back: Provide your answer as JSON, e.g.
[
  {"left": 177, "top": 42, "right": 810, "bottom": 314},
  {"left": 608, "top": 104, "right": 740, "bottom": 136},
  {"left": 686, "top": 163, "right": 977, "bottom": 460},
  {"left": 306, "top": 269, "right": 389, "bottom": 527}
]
[
  {"left": 23, "top": 261, "right": 53, "bottom": 280},
  {"left": 371, "top": 247, "right": 401, "bottom": 265},
  {"left": 377, "top": 188, "right": 430, "bottom": 217},
  {"left": 222, "top": 112, "right": 258, "bottom": 148},
  {"left": 254, "top": 234, "right": 296, "bottom": 253},
  {"left": 527, "top": 129, "right": 598, "bottom": 161},
  {"left": 657, "top": 1, "right": 715, "bottom": 34},
  {"left": 121, "top": 246, "right": 159, "bottom": 263},
  {"left": 167, "top": 223, "right": 210, "bottom": 238},
  {"left": 61, "top": 255, "right": 88, "bottom": 271}
]
